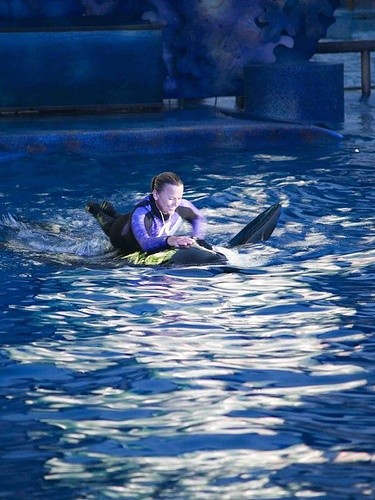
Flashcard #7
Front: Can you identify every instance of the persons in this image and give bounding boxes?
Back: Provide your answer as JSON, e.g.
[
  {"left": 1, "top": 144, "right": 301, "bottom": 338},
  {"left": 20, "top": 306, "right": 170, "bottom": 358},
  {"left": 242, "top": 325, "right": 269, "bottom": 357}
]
[{"left": 85, "top": 171, "right": 208, "bottom": 254}]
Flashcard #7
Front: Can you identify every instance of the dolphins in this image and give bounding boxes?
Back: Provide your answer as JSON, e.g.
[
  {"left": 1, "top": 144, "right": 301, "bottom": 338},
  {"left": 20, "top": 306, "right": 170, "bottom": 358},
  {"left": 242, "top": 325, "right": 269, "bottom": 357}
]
[{"left": 0, "top": 200, "right": 282, "bottom": 266}]
